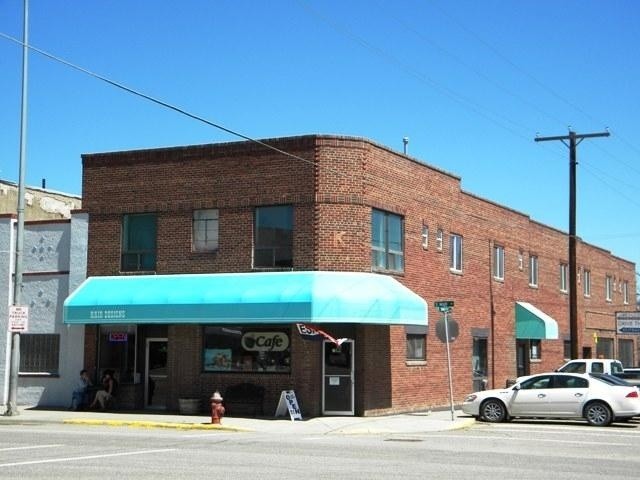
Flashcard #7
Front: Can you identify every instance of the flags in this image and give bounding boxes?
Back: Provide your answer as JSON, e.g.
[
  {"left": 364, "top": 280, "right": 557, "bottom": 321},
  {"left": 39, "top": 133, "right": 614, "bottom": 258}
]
[{"left": 296, "top": 324, "right": 348, "bottom": 348}]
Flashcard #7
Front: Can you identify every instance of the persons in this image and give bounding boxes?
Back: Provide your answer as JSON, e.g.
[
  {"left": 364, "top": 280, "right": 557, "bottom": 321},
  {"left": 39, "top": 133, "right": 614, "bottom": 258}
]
[
  {"left": 90, "top": 370, "right": 117, "bottom": 407},
  {"left": 72, "top": 370, "right": 94, "bottom": 409}
]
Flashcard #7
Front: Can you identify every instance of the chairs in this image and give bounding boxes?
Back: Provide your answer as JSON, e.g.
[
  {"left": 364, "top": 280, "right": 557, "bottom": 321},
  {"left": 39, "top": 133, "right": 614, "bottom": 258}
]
[
  {"left": 71, "top": 383, "right": 93, "bottom": 410},
  {"left": 99, "top": 368, "right": 120, "bottom": 409}
]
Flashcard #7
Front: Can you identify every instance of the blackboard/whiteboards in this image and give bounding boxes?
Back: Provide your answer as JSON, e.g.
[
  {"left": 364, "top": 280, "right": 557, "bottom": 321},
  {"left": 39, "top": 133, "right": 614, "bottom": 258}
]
[{"left": 283, "top": 390, "right": 302, "bottom": 418}]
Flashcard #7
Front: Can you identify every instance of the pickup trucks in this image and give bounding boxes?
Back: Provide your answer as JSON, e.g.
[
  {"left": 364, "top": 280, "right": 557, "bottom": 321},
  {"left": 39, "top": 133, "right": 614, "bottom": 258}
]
[{"left": 515, "top": 359, "right": 640, "bottom": 422}]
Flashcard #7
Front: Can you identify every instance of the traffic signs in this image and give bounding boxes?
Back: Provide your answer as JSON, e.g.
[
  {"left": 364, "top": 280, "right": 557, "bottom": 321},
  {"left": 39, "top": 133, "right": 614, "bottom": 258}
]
[
  {"left": 8, "top": 305, "right": 29, "bottom": 333},
  {"left": 434, "top": 301, "right": 455, "bottom": 306},
  {"left": 438, "top": 306, "right": 452, "bottom": 312}
]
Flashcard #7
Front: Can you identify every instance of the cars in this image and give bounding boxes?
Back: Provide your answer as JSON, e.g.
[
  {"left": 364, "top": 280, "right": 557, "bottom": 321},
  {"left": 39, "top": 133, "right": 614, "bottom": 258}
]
[
  {"left": 623, "top": 368, "right": 640, "bottom": 373},
  {"left": 462, "top": 372, "right": 640, "bottom": 426}
]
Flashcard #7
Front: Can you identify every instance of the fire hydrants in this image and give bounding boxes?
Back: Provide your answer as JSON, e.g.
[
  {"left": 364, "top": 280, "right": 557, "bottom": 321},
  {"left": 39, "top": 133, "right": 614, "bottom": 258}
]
[{"left": 210, "top": 391, "right": 226, "bottom": 423}]
